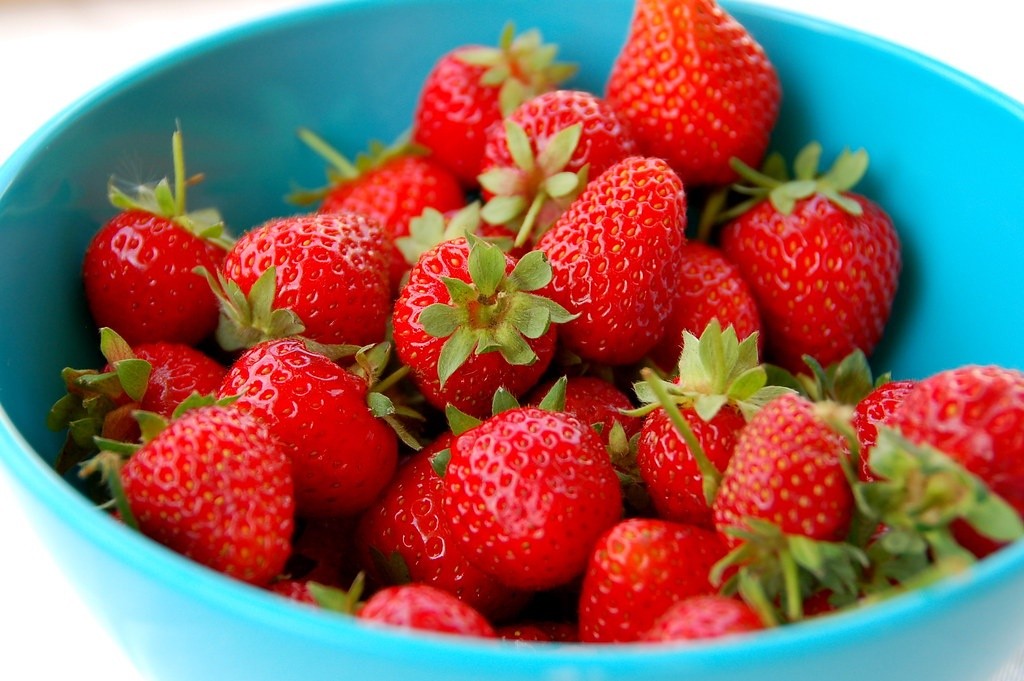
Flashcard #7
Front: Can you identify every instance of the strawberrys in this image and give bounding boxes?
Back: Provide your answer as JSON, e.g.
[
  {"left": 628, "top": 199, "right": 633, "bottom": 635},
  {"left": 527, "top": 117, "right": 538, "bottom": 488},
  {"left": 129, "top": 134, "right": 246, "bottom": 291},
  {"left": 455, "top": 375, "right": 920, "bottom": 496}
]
[{"left": 53, "top": 1, "right": 1024, "bottom": 639}]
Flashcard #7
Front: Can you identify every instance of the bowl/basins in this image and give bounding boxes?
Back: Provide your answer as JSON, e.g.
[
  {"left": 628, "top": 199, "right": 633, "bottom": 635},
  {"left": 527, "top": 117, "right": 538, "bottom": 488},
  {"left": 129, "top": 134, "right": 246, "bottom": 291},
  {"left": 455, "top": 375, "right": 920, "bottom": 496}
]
[{"left": 0, "top": 0, "right": 1024, "bottom": 681}]
